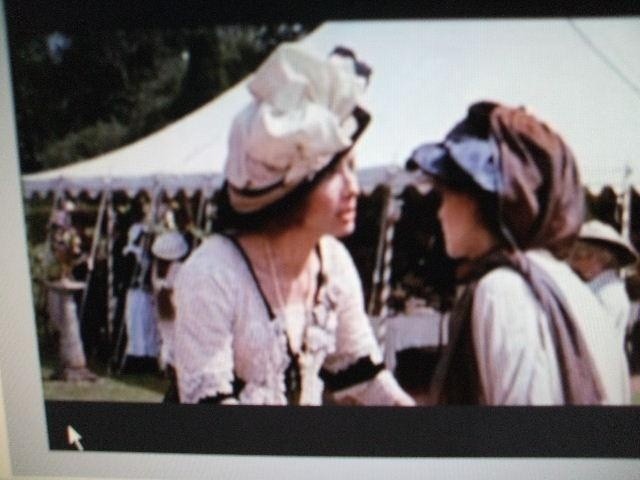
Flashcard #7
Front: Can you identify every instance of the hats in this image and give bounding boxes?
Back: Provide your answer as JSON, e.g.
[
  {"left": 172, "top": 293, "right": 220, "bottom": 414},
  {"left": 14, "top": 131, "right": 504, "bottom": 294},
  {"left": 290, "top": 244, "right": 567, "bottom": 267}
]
[
  {"left": 213, "top": 43, "right": 371, "bottom": 229},
  {"left": 579, "top": 218, "right": 640, "bottom": 266},
  {"left": 412, "top": 101, "right": 584, "bottom": 216},
  {"left": 150, "top": 233, "right": 190, "bottom": 261}
]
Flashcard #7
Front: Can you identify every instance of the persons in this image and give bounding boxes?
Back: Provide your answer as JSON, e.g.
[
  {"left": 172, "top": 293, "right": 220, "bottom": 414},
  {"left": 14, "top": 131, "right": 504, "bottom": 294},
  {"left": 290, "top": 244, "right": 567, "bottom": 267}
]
[
  {"left": 408, "top": 102, "right": 630, "bottom": 408},
  {"left": 34, "top": 204, "right": 190, "bottom": 379},
  {"left": 172, "top": 42, "right": 414, "bottom": 409}
]
